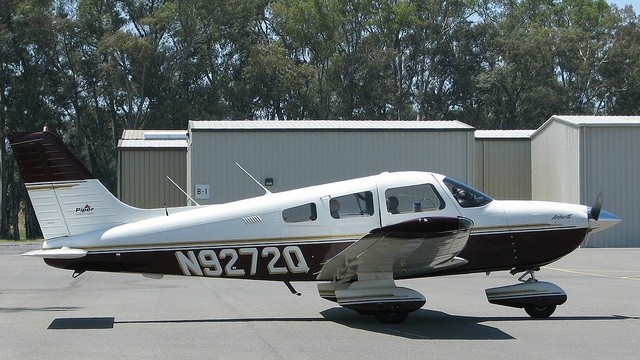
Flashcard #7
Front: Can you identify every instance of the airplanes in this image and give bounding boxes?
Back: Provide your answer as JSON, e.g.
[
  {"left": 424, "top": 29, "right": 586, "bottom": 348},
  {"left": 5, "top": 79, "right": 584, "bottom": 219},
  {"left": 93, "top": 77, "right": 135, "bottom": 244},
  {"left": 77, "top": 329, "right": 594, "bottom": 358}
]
[{"left": 5, "top": 126, "right": 624, "bottom": 322}]
